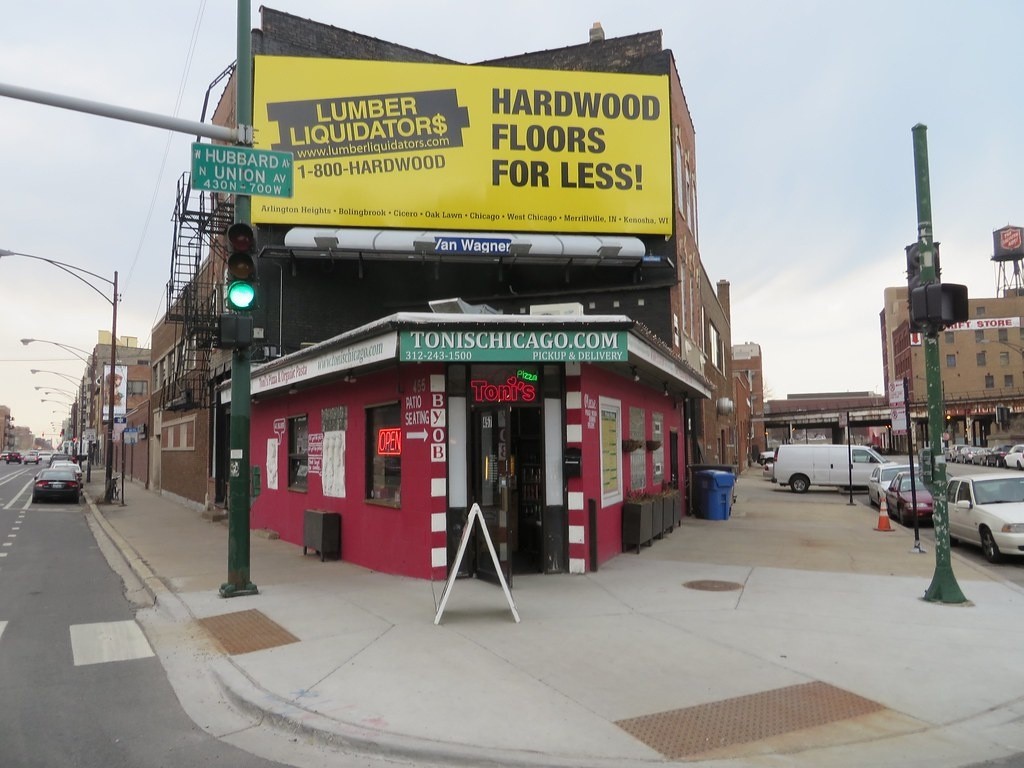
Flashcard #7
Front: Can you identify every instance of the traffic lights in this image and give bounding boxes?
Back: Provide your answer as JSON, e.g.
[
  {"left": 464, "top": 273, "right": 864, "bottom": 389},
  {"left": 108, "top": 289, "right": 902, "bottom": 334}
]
[
  {"left": 927, "top": 283, "right": 968, "bottom": 326},
  {"left": 227, "top": 223, "right": 257, "bottom": 310}
]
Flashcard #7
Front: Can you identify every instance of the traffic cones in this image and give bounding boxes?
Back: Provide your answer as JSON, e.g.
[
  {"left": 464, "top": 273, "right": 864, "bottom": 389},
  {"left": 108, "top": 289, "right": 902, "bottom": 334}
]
[{"left": 872, "top": 498, "right": 896, "bottom": 533}]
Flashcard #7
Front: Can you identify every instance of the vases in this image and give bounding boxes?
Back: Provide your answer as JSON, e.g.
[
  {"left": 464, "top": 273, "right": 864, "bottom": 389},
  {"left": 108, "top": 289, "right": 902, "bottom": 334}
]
[
  {"left": 621, "top": 489, "right": 682, "bottom": 555},
  {"left": 622, "top": 441, "right": 638, "bottom": 453},
  {"left": 646, "top": 440, "right": 662, "bottom": 452}
]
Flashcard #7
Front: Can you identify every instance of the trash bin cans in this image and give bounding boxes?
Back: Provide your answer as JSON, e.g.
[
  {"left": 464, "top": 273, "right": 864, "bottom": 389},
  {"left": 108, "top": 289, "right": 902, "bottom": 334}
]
[
  {"left": 687, "top": 462, "right": 734, "bottom": 513},
  {"left": 697, "top": 469, "right": 735, "bottom": 521}
]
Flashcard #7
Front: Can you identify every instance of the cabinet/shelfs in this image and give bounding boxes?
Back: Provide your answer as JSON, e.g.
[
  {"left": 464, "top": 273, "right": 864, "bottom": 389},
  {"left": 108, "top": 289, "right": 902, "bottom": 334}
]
[{"left": 518, "top": 463, "right": 544, "bottom": 555}]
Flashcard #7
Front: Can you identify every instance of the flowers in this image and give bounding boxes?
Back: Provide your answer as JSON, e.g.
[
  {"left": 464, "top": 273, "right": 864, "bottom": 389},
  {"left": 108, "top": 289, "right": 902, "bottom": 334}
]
[{"left": 626, "top": 478, "right": 649, "bottom": 505}]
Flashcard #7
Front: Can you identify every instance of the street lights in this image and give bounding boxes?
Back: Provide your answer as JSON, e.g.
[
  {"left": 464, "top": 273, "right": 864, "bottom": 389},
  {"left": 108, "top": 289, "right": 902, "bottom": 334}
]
[
  {"left": 36, "top": 386, "right": 78, "bottom": 465},
  {"left": 22, "top": 339, "right": 96, "bottom": 482},
  {"left": 0, "top": 248, "right": 118, "bottom": 502},
  {"left": 30, "top": 369, "right": 84, "bottom": 468}
]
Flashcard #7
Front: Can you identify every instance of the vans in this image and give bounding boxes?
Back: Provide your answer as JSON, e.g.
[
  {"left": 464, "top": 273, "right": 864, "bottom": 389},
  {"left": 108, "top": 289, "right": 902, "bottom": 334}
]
[{"left": 772, "top": 444, "right": 897, "bottom": 493}]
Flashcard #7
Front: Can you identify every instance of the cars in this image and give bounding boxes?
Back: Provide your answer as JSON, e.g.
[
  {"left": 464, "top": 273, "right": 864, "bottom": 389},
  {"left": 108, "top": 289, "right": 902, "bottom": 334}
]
[
  {"left": 38, "top": 450, "right": 55, "bottom": 460},
  {"left": 5, "top": 451, "right": 21, "bottom": 464},
  {"left": 869, "top": 464, "right": 919, "bottom": 505},
  {"left": 886, "top": 471, "right": 955, "bottom": 527},
  {"left": 944, "top": 441, "right": 1012, "bottom": 470},
  {"left": 946, "top": 473, "right": 1024, "bottom": 563},
  {"left": 0, "top": 451, "right": 8, "bottom": 460},
  {"left": 48, "top": 454, "right": 82, "bottom": 483},
  {"left": 758, "top": 450, "right": 774, "bottom": 479},
  {"left": 33, "top": 469, "right": 80, "bottom": 504},
  {"left": 1003, "top": 444, "right": 1024, "bottom": 470},
  {"left": 24, "top": 452, "right": 39, "bottom": 465}
]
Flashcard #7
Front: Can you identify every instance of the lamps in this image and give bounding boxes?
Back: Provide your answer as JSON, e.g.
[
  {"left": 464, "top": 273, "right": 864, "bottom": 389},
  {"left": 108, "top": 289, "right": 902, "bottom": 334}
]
[
  {"left": 413, "top": 241, "right": 436, "bottom": 265},
  {"left": 684, "top": 391, "right": 689, "bottom": 402},
  {"left": 314, "top": 237, "right": 339, "bottom": 264},
  {"left": 631, "top": 365, "right": 640, "bottom": 381},
  {"left": 662, "top": 382, "right": 669, "bottom": 396},
  {"left": 593, "top": 247, "right": 621, "bottom": 269},
  {"left": 343, "top": 370, "right": 357, "bottom": 383},
  {"left": 289, "top": 383, "right": 298, "bottom": 394},
  {"left": 509, "top": 244, "right": 533, "bottom": 269},
  {"left": 251, "top": 396, "right": 260, "bottom": 404}
]
